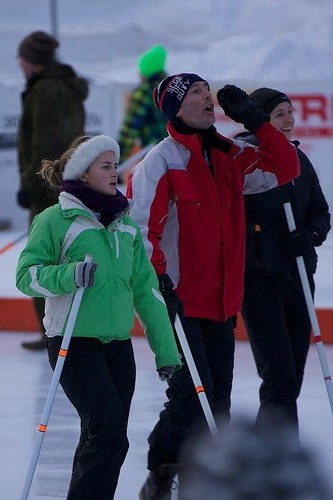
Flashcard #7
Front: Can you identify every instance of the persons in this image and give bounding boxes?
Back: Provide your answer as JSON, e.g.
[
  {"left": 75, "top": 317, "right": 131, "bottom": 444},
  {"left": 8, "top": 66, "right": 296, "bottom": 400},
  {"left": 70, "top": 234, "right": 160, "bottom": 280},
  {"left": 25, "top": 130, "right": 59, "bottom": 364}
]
[
  {"left": 16, "top": 134, "right": 183, "bottom": 500},
  {"left": 127, "top": 73, "right": 302, "bottom": 500},
  {"left": 15, "top": 32, "right": 90, "bottom": 351},
  {"left": 115, "top": 44, "right": 172, "bottom": 165},
  {"left": 230, "top": 88, "right": 332, "bottom": 456}
]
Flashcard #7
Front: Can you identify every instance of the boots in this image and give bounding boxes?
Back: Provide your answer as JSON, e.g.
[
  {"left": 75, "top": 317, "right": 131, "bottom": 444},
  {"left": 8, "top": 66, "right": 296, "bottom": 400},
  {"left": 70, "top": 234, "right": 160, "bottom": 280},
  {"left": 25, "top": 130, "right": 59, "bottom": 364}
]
[{"left": 139, "top": 463, "right": 184, "bottom": 500}]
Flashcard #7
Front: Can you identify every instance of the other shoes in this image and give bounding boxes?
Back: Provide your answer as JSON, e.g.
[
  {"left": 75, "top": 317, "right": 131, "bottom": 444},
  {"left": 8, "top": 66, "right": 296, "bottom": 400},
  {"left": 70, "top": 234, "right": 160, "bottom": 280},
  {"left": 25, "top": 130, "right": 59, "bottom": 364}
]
[{"left": 22, "top": 340, "right": 46, "bottom": 350}]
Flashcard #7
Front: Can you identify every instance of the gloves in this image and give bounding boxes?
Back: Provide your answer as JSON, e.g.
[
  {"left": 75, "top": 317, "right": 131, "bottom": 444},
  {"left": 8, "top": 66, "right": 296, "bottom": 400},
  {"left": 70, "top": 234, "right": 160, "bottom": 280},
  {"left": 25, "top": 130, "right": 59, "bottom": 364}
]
[
  {"left": 286, "top": 227, "right": 318, "bottom": 260},
  {"left": 17, "top": 189, "right": 34, "bottom": 209},
  {"left": 156, "top": 274, "right": 184, "bottom": 335},
  {"left": 75, "top": 262, "right": 98, "bottom": 288},
  {"left": 159, "top": 366, "right": 176, "bottom": 381},
  {"left": 216, "top": 84, "right": 271, "bottom": 135}
]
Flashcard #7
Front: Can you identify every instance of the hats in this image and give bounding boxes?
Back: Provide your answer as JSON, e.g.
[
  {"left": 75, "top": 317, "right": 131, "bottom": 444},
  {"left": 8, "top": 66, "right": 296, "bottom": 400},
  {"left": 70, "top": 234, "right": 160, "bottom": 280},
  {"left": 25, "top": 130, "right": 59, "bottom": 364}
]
[
  {"left": 18, "top": 32, "right": 59, "bottom": 65},
  {"left": 139, "top": 46, "right": 168, "bottom": 76},
  {"left": 153, "top": 73, "right": 210, "bottom": 121},
  {"left": 249, "top": 87, "right": 292, "bottom": 118}
]
